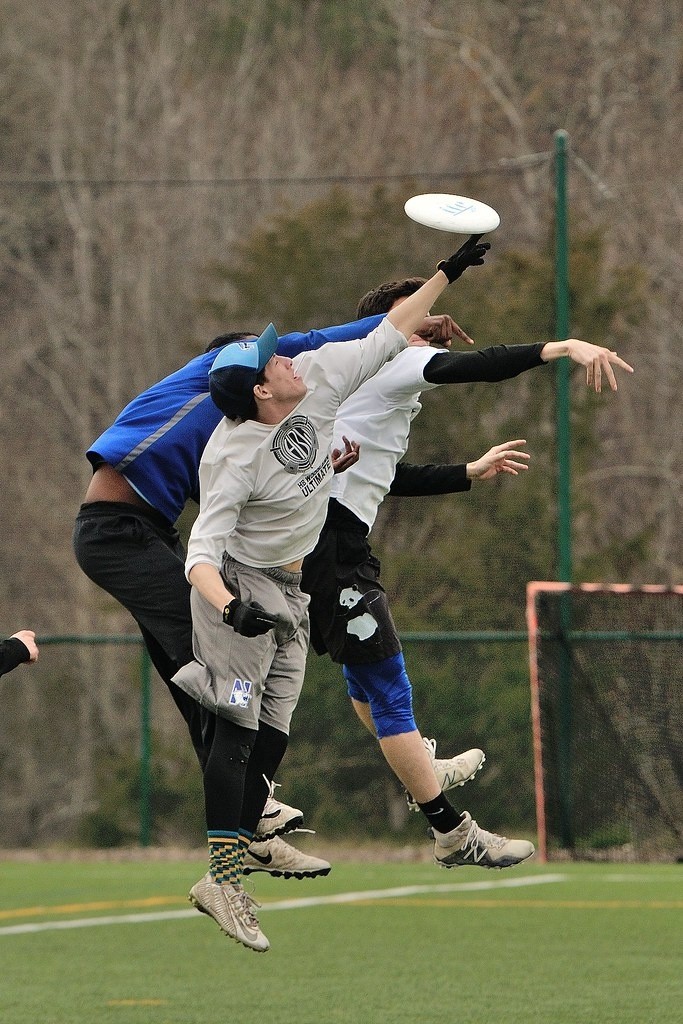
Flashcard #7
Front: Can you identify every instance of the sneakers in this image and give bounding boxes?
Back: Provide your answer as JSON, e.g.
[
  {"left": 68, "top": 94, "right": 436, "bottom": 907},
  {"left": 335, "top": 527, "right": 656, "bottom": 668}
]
[
  {"left": 427, "top": 810, "right": 535, "bottom": 870},
  {"left": 404, "top": 737, "right": 486, "bottom": 813},
  {"left": 243, "top": 828, "right": 331, "bottom": 881},
  {"left": 249, "top": 773, "right": 304, "bottom": 844},
  {"left": 188, "top": 871, "right": 270, "bottom": 953}
]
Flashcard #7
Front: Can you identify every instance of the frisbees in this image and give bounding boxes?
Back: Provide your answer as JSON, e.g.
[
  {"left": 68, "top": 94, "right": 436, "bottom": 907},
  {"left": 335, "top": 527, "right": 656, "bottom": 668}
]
[{"left": 403, "top": 192, "right": 501, "bottom": 235}]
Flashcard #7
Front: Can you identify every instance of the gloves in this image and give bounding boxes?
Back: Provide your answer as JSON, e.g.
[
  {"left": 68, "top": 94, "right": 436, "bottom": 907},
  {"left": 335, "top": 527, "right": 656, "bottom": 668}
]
[
  {"left": 436, "top": 232, "right": 491, "bottom": 285},
  {"left": 222, "top": 598, "right": 279, "bottom": 638}
]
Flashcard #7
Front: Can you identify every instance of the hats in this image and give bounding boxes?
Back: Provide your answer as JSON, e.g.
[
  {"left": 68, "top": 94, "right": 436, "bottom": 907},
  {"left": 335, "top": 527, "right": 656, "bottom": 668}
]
[{"left": 208, "top": 322, "right": 278, "bottom": 421}]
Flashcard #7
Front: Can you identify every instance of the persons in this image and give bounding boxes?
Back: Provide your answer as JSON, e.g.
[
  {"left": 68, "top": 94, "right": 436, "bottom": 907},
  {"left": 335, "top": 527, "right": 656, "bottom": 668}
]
[
  {"left": 73, "top": 315, "right": 474, "bottom": 880},
  {"left": 298, "top": 274, "right": 634, "bottom": 869},
  {"left": 0, "top": 630, "right": 39, "bottom": 678},
  {"left": 172, "top": 231, "right": 491, "bottom": 952}
]
